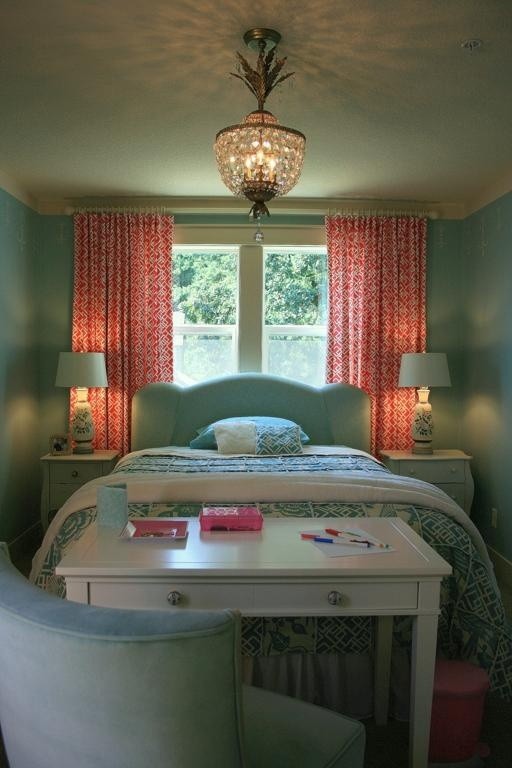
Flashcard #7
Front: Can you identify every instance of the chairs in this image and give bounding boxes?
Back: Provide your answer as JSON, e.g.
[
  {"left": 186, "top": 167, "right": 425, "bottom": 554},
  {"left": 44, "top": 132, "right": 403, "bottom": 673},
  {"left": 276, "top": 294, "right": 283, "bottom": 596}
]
[{"left": 0, "top": 542, "right": 366, "bottom": 768}]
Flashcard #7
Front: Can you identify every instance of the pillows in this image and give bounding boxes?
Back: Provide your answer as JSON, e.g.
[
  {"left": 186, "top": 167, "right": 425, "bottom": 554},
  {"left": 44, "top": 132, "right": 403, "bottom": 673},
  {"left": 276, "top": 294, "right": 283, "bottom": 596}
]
[{"left": 189, "top": 415, "right": 309, "bottom": 456}]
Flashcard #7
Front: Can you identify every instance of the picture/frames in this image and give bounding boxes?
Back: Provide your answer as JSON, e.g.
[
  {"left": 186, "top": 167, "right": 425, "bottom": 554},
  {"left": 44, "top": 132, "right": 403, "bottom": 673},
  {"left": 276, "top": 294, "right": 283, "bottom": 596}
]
[{"left": 48, "top": 433, "right": 72, "bottom": 456}]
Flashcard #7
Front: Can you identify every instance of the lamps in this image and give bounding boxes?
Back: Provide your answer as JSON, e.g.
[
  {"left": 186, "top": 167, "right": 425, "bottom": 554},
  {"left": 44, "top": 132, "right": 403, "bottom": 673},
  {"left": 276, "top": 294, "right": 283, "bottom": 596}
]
[
  {"left": 399, "top": 352, "right": 452, "bottom": 454},
  {"left": 214, "top": 28, "right": 306, "bottom": 243},
  {"left": 55, "top": 352, "right": 109, "bottom": 454}
]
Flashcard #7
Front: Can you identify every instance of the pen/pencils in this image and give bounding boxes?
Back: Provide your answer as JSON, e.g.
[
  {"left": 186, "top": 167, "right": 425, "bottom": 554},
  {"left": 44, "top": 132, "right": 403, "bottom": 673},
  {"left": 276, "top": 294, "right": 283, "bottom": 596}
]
[{"left": 300, "top": 529, "right": 389, "bottom": 548}]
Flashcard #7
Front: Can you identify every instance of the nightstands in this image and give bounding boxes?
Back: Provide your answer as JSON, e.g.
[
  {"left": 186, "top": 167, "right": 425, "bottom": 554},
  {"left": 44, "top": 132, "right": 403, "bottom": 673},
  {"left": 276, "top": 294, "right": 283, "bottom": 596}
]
[
  {"left": 39, "top": 450, "right": 119, "bottom": 532},
  {"left": 378, "top": 450, "right": 475, "bottom": 518}
]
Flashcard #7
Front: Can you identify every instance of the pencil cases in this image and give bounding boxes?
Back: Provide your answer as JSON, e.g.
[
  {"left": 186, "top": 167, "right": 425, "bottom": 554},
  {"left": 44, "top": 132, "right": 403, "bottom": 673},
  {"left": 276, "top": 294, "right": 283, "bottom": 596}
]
[{"left": 199, "top": 507, "right": 263, "bottom": 531}]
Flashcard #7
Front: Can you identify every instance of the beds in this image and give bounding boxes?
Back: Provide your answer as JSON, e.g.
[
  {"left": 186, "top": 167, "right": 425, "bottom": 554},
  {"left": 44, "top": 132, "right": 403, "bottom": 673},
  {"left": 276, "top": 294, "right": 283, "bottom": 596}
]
[{"left": 30, "top": 372, "right": 512, "bottom": 720}]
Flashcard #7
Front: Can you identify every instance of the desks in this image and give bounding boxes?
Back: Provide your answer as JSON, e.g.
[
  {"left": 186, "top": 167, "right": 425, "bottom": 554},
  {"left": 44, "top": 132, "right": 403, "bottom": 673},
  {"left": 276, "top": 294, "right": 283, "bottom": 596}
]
[{"left": 55, "top": 518, "right": 453, "bottom": 768}]
